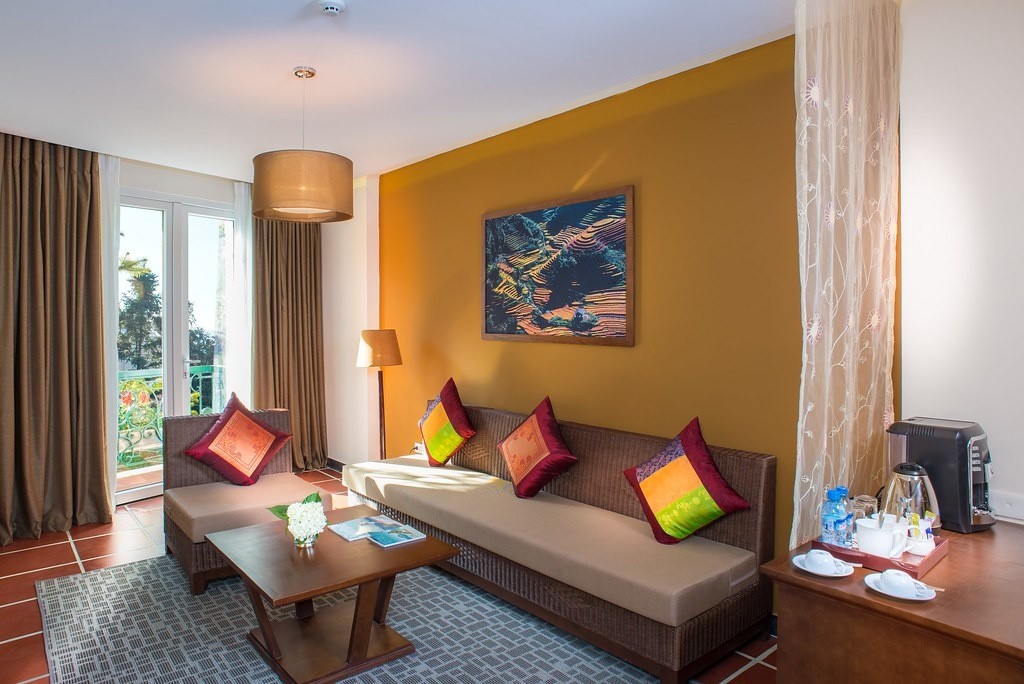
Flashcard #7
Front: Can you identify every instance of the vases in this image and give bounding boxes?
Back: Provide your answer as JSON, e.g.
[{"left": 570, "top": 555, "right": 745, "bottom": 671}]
[{"left": 293, "top": 534, "right": 316, "bottom": 547}]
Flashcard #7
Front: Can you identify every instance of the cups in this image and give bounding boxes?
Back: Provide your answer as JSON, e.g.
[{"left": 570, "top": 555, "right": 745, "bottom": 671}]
[
  {"left": 871, "top": 512, "right": 922, "bottom": 558},
  {"left": 855, "top": 495, "right": 878, "bottom": 518},
  {"left": 875, "top": 568, "right": 928, "bottom": 598},
  {"left": 856, "top": 518, "right": 905, "bottom": 559},
  {"left": 906, "top": 534, "right": 936, "bottom": 556},
  {"left": 850, "top": 500, "right": 866, "bottom": 543},
  {"left": 805, "top": 548, "right": 845, "bottom": 574}
]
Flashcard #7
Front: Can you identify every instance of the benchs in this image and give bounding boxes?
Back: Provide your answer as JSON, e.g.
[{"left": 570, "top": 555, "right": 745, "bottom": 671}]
[{"left": 341, "top": 399, "right": 778, "bottom": 684}]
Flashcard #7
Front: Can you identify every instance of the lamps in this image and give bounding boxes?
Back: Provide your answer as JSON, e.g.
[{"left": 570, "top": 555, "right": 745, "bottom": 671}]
[
  {"left": 251, "top": 66, "right": 354, "bottom": 223},
  {"left": 354, "top": 329, "right": 403, "bottom": 460}
]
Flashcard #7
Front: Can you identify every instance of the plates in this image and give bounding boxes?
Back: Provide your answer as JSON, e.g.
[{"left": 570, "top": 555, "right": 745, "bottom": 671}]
[
  {"left": 864, "top": 573, "right": 936, "bottom": 601},
  {"left": 792, "top": 554, "right": 854, "bottom": 577}
]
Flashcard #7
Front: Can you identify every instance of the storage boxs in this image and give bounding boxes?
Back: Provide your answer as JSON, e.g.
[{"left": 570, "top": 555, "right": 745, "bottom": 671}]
[{"left": 813, "top": 534, "right": 949, "bottom": 582}]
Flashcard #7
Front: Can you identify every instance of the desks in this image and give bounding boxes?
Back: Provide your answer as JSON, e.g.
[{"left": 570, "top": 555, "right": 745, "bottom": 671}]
[{"left": 759, "top": 518, "right": 1024, "bottom": 684}]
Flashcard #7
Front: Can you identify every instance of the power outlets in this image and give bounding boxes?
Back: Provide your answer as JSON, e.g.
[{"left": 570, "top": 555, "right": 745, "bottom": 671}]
[{"left": 414, "top": 442, "right": 421, "bottom": 454}]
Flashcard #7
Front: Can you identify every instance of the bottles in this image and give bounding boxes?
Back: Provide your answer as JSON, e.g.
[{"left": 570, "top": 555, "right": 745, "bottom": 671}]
[
  {"left": 836, "top": 485, "right": 855, "bottom": 548},
  {"left": 821, "top": 489, "right": 847, "bottom": 548}
]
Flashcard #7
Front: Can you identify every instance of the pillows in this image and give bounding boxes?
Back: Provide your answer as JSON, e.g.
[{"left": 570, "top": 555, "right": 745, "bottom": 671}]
[
  {"left": 623, "top": 416, "right": 751, "bottom": 545},
  {"left": 417, "top": 377, "right": 476, "bottom": 467},
  {"left": 496, "top": 394, "right": 578, "bottom": 498},
  {"left": 184, "top": 392, "right": 293, "bottom": 486}
]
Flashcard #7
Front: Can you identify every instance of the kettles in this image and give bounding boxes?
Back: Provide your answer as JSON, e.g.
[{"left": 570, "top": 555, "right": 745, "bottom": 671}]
[{"left": 880, "top": 461, "right": 940, "bottom": 537}]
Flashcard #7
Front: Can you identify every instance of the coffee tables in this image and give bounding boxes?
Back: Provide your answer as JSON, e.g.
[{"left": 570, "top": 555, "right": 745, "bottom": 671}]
[{"left": 204, "top": 504, "right": 460, "bottom": 684}]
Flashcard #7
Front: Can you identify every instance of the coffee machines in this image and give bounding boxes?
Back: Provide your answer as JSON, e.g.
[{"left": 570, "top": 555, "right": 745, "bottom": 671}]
[{"left": 886, "top": 416, "right": 996, "bottom": 533}]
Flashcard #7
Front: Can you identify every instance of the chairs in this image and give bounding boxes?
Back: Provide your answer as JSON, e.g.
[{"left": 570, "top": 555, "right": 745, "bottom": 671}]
[{"left": 161, "top": 408, "right": 334, "bottom": 595}]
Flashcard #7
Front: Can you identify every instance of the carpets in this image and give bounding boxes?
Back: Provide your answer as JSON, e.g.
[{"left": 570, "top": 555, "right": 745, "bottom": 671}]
[{"left": 34, "top": 554, "right": 700, "bottom": 683}]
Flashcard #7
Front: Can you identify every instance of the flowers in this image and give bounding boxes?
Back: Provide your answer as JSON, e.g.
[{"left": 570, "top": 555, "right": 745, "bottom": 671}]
[{"left": 266, "top": 490, "right": 332, "bottom": 545}]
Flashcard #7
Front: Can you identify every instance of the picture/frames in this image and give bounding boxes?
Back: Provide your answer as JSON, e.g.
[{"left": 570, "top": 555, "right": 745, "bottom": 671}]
[{"left": 479, "top": 183, "right": 635, "bottom": 349}]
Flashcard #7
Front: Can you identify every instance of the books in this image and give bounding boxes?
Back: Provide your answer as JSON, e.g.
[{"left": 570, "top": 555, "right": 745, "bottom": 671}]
[
  {"left": 366, "top": 525, "right": 426, "bottom": 547},
  {"left": 327, "top": 515, "right": 404, "bottom": 542}
]
